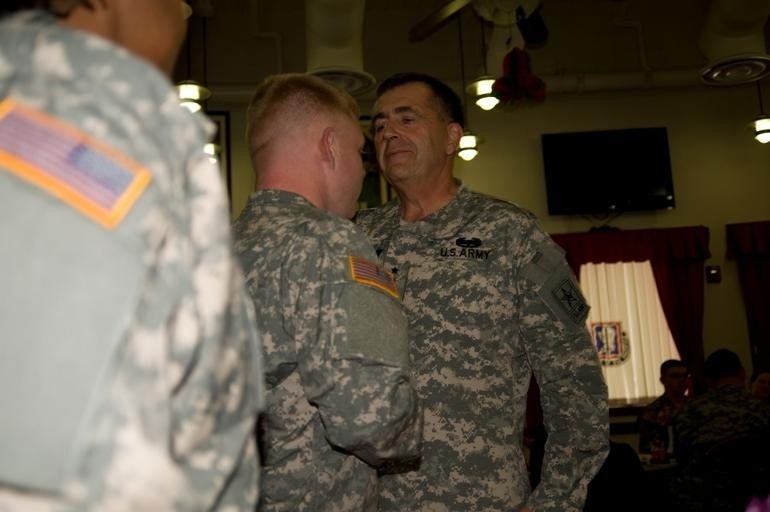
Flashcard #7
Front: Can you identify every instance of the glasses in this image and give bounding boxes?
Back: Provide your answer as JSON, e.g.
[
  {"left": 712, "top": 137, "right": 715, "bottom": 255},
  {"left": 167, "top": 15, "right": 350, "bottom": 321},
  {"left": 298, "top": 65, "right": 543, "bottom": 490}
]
[{"left": 670, "top": 373, "right": 689, "bottom": 379}]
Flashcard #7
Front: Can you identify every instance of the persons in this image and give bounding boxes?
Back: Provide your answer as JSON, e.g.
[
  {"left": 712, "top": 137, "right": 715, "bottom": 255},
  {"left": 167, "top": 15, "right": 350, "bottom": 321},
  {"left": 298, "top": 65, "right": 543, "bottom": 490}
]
[
  {"left": 225, "top": 71, "right": 422, "bottom": 512},
  {"left": 0, "top": 0, "right": 267, "bottom": 512},
  {"left": 528, "top": 350, "right": 770, "bottom": 512},
  {"left": 343, "top": 74, "right": 610, "bottom": 512}
]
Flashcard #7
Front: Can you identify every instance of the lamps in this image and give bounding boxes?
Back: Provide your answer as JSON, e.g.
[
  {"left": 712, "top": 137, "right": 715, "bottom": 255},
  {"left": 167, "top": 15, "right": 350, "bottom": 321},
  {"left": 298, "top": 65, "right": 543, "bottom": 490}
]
[
  {"left": 170, "top": 22, "right": 212, "bottom": 103},
  {"left": 745, "top": 79, "right": 769, "bottom": 143},
  {"left": 445, "top": 0, "right": 499, "bottom": 162}
]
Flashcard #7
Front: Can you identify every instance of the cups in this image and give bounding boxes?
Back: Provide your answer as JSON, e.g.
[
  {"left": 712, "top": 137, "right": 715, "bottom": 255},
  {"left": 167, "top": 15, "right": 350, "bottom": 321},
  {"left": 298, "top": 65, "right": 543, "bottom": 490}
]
[{"left": 650, "top": 440, "right": 667, "bottom": 464}]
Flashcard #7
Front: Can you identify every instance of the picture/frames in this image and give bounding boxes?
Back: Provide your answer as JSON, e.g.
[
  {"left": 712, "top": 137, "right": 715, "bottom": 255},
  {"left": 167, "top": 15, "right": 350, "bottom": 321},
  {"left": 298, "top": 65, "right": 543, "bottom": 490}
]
[
  {"left": 209, "top": 111, "right": 230, "bottom": 214},
  {"left": 354, "top": 116, "right": 391, "bottom": 213}
]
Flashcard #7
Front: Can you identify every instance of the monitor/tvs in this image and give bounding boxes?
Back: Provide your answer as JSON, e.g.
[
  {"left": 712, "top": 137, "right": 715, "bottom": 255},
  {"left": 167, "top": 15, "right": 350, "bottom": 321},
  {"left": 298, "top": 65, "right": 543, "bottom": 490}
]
[{"left": 542, "top": 127, "right": 676, "bottom": 216}]
